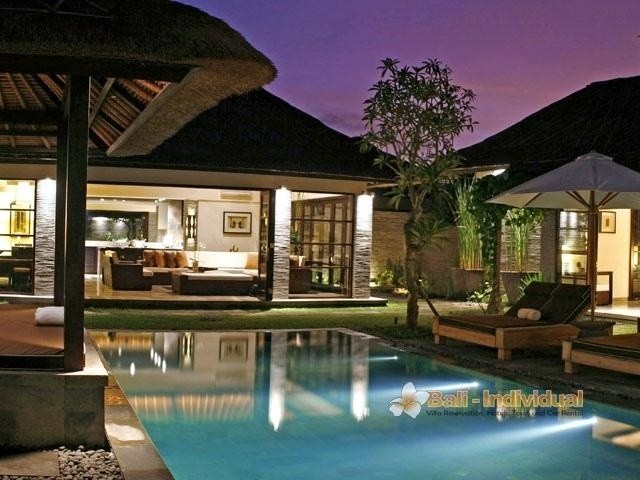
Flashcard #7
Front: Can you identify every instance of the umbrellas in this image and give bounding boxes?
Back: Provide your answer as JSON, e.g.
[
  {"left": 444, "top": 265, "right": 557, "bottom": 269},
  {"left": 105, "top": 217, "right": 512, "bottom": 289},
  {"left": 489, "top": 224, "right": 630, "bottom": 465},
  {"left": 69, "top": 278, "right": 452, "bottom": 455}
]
[{"left": 486, "top": 150, "right": 640, "bottom": 321}]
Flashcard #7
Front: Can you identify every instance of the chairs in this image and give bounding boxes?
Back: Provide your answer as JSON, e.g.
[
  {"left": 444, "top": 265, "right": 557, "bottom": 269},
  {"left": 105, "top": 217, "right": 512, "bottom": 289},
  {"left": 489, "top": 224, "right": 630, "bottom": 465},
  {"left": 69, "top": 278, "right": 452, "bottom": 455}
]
[
  {"left": 562, "top": 333, "right": 639, "bottom": 382},
  {"left": 102, "top": 250, "right": 153, "bottom": 290},
  {"left": 430, "top": 281, "right": 593, "bottom": 362}
]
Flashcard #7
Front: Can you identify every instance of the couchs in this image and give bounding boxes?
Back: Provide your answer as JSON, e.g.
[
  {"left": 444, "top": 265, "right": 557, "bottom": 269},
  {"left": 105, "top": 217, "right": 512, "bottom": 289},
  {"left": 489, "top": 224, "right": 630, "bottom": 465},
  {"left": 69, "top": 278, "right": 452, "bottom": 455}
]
[{"left": 144, "top": 247, "right": 309, "bottom": 294}]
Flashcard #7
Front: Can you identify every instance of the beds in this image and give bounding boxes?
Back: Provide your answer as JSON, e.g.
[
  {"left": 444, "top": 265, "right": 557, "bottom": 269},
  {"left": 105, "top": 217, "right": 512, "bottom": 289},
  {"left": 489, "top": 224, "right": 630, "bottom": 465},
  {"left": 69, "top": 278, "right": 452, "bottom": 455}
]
[{"left": 172, "top": 272, "right": 253, "bottom": 296}]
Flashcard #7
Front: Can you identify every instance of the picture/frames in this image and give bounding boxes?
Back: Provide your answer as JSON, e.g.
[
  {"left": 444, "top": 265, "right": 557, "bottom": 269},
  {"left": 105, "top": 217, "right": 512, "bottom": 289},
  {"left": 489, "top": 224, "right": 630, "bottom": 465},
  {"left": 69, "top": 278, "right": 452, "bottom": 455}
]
[
  {"left": 601, "top": 211, "right": 616, "bottom": 233},
  {"left": 224, "top": 212, "right": 252, "bottom": 235},
  {"left": 218, "top": 337, "right": 248, "bottom": 363}
]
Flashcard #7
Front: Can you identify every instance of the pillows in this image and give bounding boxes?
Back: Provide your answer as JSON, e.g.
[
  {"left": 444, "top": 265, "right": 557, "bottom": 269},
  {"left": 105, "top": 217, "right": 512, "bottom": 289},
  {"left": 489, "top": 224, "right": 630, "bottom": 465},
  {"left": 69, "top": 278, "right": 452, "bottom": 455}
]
[
  {"left": 165, "top": 251, "right": 176, "bottom": 267},
  {"left": 155, "top": 251, "right": 164, "bottom": 267},
  {"left": 176, "top": 251, "right": 188, "bottom": 268},
  {"left": 145, "top": 251, "right": 155, "bottom": 267}
]
